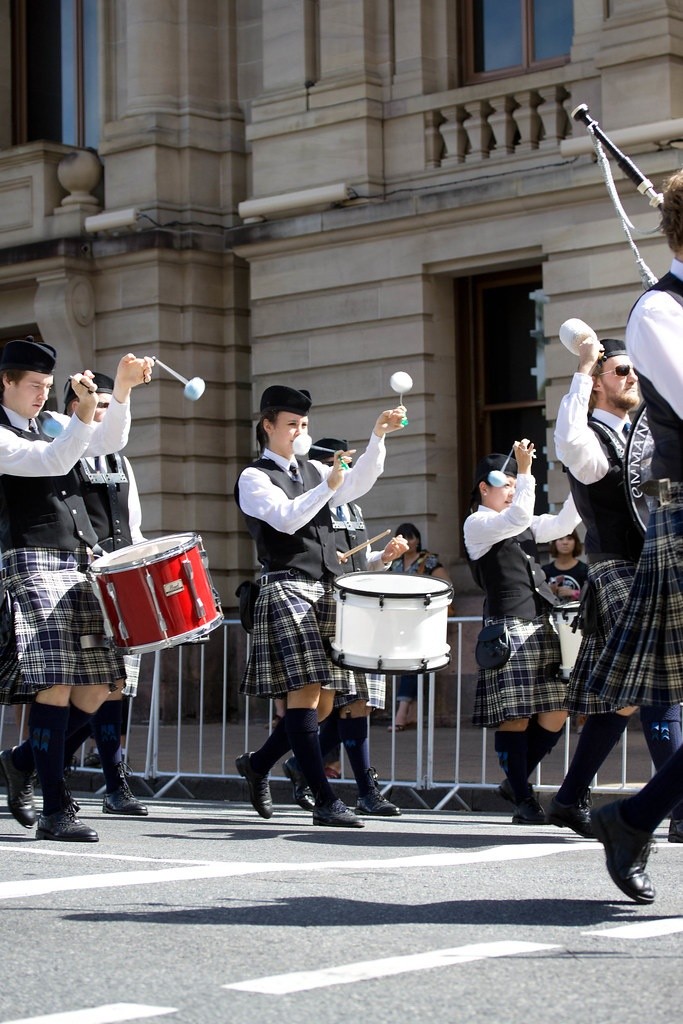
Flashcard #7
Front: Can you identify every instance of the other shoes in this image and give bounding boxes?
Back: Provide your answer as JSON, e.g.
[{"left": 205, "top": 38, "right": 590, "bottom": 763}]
[{"left": 265, "top": 715, "right": 282, "bottom": 728}]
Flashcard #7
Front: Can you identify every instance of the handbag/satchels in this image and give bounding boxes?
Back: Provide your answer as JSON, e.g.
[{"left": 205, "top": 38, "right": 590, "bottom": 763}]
[
  {"left": 235, "top": 580, "right": 259, "bottom": 633},
  {"left": 475, "top": 623, "right": 511, "bottom": 670}
]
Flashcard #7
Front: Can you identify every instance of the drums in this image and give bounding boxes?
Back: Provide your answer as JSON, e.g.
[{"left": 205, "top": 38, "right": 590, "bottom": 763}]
[
  {"left": 87, "top": 531, "right": 225, "bottom": 656},
  {"left": 622, "top": 398, "right": 657, "bottom": 537},
  {"left": 552, "top": 601, "right": 584, "bottom": 680},
  {"left": 330, "top": 569, "right": 455, "bottom": 677}
]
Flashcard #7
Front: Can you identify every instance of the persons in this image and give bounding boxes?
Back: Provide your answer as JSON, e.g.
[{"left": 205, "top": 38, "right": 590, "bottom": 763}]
[
  {"left": 1, "top": 336, "right": 153, "bottom": 842},
  {"left": 462, "top": 171, "right": 683, "bottom": 902},
  {"left": 233, "top": 384, "right": 454, "bottom": 828}
]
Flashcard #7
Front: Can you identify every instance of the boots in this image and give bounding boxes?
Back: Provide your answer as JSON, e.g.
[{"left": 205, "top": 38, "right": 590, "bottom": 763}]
[
  {"left": 283, "top": 757, "right": 316, "bottom": 811},
  {"left": 354, "top": 767, "right": 401, "bottom": 815}
]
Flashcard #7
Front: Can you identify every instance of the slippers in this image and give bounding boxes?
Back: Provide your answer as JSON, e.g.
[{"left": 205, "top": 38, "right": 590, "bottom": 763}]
[
  {"left": 388, "top": 724, "right": 406, "bottom": 731},
  {"left": 325, "top": 768, "right": 341, "bottom": 779}
]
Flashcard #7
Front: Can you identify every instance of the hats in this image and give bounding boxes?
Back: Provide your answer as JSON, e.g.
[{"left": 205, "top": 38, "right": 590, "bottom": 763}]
[
  {"left": 261, "top": 386, "right": 312, "bottom": 416},
  {"left": 471, "top": 453, "right": 518, "bottom": 493},
  {"left": 64, "top": 371, "right": 115, "bottom": 405},
  {"left": 0, "top": 336, "right": 56, "bottom": 374},
  {"left": 599, "top": 339, "right": 627, "bottom": 357},
  {"left": 308, "top": 438, "right": 348, "bottom": 461}
]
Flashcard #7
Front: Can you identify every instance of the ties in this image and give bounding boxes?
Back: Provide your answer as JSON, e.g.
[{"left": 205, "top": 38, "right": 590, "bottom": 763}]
[
  {"left": 95, "top": 456, "right": 102, "bottom": 473},
  {"left": 290, "top": 462, "right": 302, "bottom": 483},
  {"left": 337, "top": 505, "right": 347, "bottom": 521},
  {"left": 28, "top": 419, "right": 38, "bottom": 435}
]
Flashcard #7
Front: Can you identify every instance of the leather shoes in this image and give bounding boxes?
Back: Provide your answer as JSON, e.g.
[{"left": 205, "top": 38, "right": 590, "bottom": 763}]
[
  {"left": 77, "top": 751, "right": 103, "bottom": 771},
  {"left": 36, "top": 803, "right": 99, "bottom": 842},
  {"left": 496, "top": 780, "right": 544, "bottom": 815},
  {"left": 513, "top": 797, "right": 547, "bottom": 824},
  {"left": 235, "top": 751, "right": 273, "bottom": 819},
  {"left": 0, "top": 746, "right": 40, "bottom": 828},
  {"left": 589, "top": 798, "right": 657, "bottom": 903},
  {"left": 102, "top": 783, "right": 148, "bottom": 815},
  {"left": 547, "top": 789, "right": 596, "bottom": 837},
  {"left": 313, "top": 798, "right": 364, "bottom": 827},
  {"left": 667, "top": 819, "right": 683, "bottom": 842}
]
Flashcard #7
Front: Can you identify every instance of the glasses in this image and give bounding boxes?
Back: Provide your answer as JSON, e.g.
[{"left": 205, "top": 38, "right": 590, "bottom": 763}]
[
  {"left": 97, "top": 402, "right": 109, "bottom": 408},
  {"left": 591, "top": 365, "right": 638, "bottom": 377},
  {"left": 322, "top": 461, "right": 334, "bottom": 467}
]
